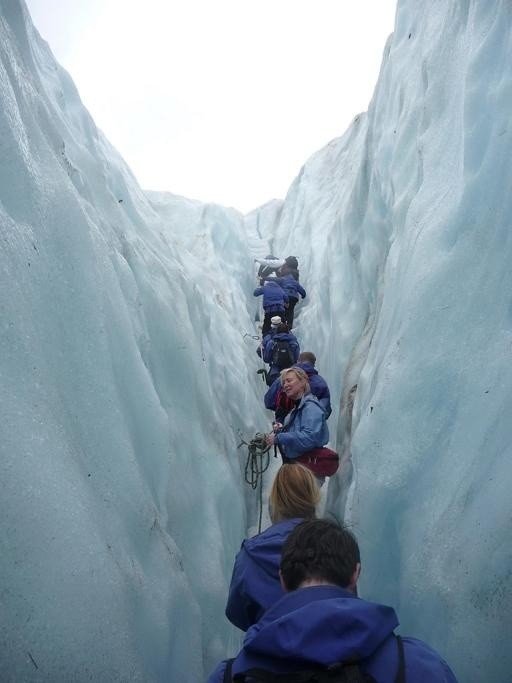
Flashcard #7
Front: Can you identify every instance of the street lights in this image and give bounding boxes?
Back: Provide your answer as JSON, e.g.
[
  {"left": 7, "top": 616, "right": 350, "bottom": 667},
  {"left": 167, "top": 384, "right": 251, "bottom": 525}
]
[{"left": 300, "top": 445, "right": 340, "bottom": 477}]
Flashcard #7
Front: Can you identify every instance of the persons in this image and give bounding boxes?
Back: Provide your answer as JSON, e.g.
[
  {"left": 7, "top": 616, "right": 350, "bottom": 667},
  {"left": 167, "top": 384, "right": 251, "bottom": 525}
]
[
  {"left": 265, "top": 366, "right": 338, "bottom": 487},
  {"left": 204, "top": 516, "right": 457, "bottom": 681},
  {"left": 265, "top": 351, "right": 332, "bottom": 421},
  {"left": 251, "top": 253, "right": 307, "bottom": 370},
  {"left": 225, "top": 462, "right": 359, "bottom": 634}
]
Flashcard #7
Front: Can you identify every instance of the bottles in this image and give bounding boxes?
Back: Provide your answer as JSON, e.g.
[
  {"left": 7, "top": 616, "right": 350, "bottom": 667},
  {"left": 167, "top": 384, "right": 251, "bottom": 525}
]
[{"left": 270, "top": 316, "right": 283, "bottom": 328}]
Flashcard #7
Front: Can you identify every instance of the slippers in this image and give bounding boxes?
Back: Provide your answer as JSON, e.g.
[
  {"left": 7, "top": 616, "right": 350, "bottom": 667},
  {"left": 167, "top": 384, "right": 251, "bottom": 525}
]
[
  {"left": 272, "top": 341, "right": 295, "bottom": 369},
  {"left": 275, "top": 388, "right": 297, "bottom": 425},
  {"left": 285, "top": 256, "right": 298, "bottom": 268}
]
